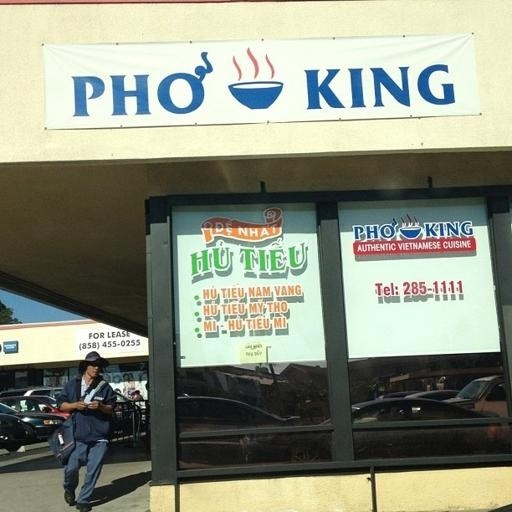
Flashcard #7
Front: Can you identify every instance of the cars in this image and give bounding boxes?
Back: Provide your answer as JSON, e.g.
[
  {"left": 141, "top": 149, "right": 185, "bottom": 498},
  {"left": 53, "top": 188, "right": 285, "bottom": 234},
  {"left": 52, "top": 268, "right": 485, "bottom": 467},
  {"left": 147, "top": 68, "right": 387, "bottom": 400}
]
[
  {"left": 0, "top": 413, "right": 26, "bottom": 452},
  {"left": 0, "top": 388, "right": 27, "bottom": 407},
  {"left": 176, "top": 396, "right": 299, "bottom": 464},
  {"left": 0, "top": 403, "right": 66, "bottom": 445},
  {"left": 378, "top": 390, "right": 459, "bottom": 417},
  {"left": 264, "top": 394, "right": 501, "bottom": 462}
]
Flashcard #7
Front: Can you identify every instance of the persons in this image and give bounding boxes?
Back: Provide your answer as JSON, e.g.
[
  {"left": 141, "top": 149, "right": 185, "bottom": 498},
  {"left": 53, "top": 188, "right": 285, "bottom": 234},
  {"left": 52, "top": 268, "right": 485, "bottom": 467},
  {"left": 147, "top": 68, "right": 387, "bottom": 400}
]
[
  {"left": 55, "top": 350, "right": 119, "bottom": 512},
  {"left": 102, "top": 369, "right": 149, "bottom": 406}
]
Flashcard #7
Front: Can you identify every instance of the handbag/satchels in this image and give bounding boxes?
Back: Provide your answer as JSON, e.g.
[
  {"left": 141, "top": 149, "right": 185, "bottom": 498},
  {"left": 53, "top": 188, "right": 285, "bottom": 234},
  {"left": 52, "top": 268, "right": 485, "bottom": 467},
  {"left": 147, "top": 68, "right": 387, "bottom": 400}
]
[{"left": 47, "top": 416, "right": 76, "bottom": 465}]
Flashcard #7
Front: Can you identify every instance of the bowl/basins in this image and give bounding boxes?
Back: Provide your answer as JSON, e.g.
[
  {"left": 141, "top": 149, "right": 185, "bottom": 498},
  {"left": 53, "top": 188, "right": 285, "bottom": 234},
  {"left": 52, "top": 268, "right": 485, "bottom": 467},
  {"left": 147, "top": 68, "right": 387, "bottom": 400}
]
[
  {"left": 227, "top": 82, "right": 283, "bottom": 109},
  {"left": 399, "top": 226, "right": 423, "bottom": 239}
]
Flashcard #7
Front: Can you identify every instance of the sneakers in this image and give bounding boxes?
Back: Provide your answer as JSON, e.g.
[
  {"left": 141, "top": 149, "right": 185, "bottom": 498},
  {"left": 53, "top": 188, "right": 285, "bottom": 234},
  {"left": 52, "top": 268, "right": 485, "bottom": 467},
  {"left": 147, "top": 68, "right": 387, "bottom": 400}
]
[
  {"left": 64, "top": 487, "right": 75, "bottom": 504},
  {"left": 75, "top": 502, "right": 92, "bottom": 511}
]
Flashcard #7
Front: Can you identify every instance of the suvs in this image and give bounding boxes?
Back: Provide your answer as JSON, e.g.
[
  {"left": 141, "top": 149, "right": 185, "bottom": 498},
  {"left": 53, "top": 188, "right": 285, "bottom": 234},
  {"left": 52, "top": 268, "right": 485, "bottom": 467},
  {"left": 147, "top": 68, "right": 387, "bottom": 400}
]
[{"left": 0, "top": 395, "right": 72, "bottom": 421}]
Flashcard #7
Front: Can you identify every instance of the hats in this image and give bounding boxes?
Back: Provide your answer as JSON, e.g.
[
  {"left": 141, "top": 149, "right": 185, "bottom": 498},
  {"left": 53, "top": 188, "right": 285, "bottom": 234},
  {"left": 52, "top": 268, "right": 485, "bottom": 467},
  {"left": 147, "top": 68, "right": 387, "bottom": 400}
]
[{"left": 79, "top": 351, "right": 111, "bottom": 374}]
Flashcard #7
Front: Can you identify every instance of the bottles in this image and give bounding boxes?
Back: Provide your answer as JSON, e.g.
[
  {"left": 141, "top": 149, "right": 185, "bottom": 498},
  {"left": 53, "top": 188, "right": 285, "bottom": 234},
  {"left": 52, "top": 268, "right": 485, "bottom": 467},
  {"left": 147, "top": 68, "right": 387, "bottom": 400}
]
[{"left": 57, "top": 432, "right": 65, "bottom": 446}]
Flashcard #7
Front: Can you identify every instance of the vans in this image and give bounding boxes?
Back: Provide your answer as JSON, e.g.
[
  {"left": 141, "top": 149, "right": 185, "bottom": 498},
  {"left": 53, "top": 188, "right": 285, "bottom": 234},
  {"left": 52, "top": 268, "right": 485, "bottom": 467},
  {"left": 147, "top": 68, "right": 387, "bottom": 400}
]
[
  {"left": 458, "top": 375, "right": 508, "bottom": 416},
  {"left": 19, "top": 387, "right": 139, "bottom": 421}
]
